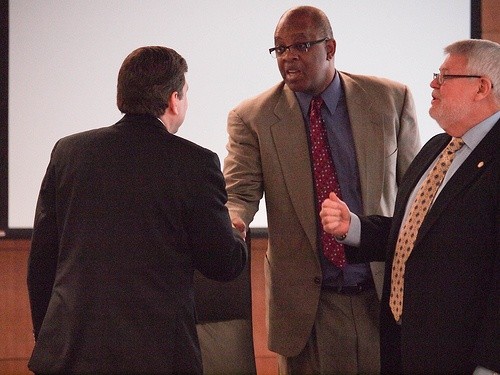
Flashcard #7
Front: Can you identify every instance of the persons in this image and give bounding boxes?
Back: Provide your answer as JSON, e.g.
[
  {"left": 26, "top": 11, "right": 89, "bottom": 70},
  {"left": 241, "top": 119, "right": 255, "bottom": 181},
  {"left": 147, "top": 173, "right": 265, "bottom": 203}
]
[
  {"left": 24, "top": 43, "right": 251, "bottom": 375},
  {"left": 217, "top": 2, "right": 425, "bottom": 375},
  {"left": 320, "top": 35, "right": 500, "bottom": 375}
]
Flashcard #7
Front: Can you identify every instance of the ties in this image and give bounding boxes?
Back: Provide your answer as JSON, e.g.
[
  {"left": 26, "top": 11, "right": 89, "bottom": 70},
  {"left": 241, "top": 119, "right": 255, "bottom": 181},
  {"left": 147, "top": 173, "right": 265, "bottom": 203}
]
[
  {"left": 389, "top": 136, "right": 464, "bottom": 326},
  {"left": 309, "top": 97, "right": 347, "bottom": 270}
]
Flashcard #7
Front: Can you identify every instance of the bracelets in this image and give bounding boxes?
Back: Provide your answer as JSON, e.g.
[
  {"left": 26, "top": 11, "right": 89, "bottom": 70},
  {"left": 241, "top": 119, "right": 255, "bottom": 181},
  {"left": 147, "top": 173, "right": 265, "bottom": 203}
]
[{"left": 336, "top": 233, "right": 348, "bottom": 240}]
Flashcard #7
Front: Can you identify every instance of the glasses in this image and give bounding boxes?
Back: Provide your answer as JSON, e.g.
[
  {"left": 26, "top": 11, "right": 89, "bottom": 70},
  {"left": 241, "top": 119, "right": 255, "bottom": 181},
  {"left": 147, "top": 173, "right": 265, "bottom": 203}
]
[
  {"left": 269, "top": 37, "right": 330, "bottom": 58},
  {"left": 432, "top": 72, "right": 494, "bottom": 89}
]
